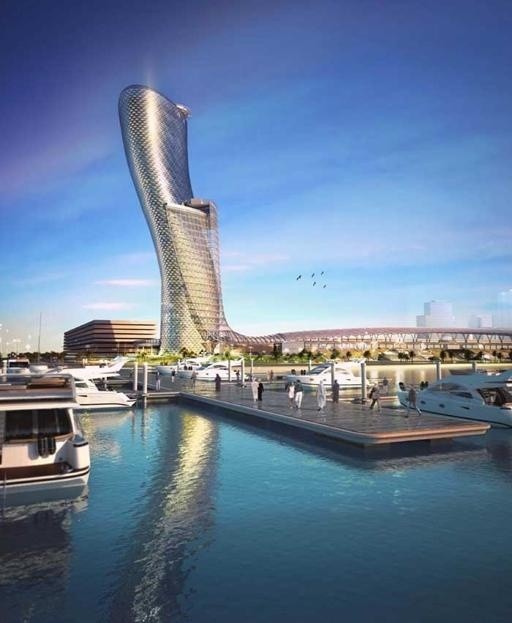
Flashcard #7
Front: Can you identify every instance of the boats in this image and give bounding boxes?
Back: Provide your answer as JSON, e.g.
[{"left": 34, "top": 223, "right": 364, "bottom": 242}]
[
  {"left": 0, "top": 356, "right": 137, "bottom": 508},
  {"left": 397, "top": 367, "right": 512, "bottom": 430},
  {"left": 286, "top": 363, "right": 370, "bottom": 390},
  {"left": 156, "top": 357, "right": 250, "bottom": 382}
]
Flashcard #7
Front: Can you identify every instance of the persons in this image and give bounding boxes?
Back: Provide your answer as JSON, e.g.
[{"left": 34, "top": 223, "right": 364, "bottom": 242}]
[
  {"left": 403, "top": 385, "right": 421, "bottom": 417},
  {"left": 332, "top": 378, "right": 339, "bottom": 403},
  {"left": 258, "top": 381, "right": 265, "bottom": 400},
  {"left": 252, "top": 378, "right": 259, "bottom": 401},
  {"left": 214, "top": 372, "right": 222, "bottom": 391},
  {"left": 155, "top": 370, "right": 161, "bottom": 391},
  {"left": 171, "top": 367, "right": 176, "bottom": 383},
  {"left": 369, "top": 380, "right": 381, "bottom": 411},
  {"left": 315, "top": 379, "right": 326, "bottom": 410},
  {"left": 382, "top": 376, "right": 388, "bottom": 395},
  {"left": 286, "top": 379, "right": 296, "bottom": 409},
  {"left": 295, "top": 378, "right": 303, "bottom": 408},
  {"left": 191, "top": 370, "right": 197, "bottom": 387}
]
